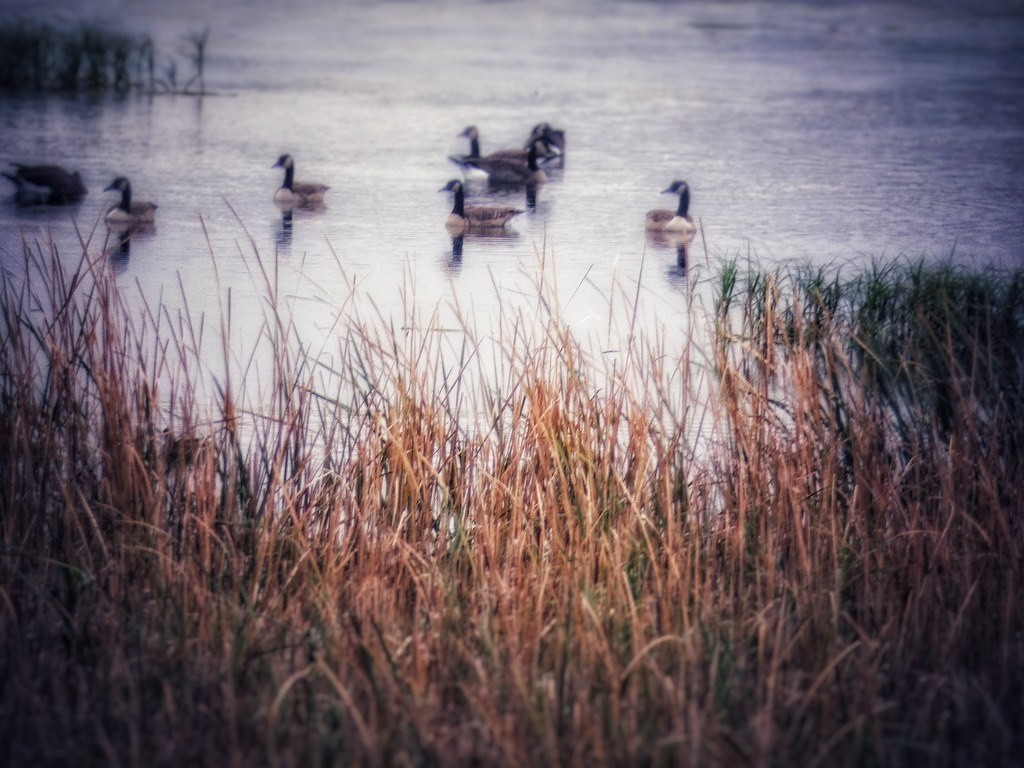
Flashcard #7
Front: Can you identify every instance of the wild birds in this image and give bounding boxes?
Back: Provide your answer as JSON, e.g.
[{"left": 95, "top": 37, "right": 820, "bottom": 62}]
[
  {"left": 0, "top": 163, "right": 159, "bottom": 221},
  {"left": 275, "top": 201, "right": 327, "bottom": 244},
  {"left": 445, "top": 226, "right": 520, "bottom": 271},
  {"left": 106, "top": 221, "right": 156, "bottom": 263},
  {"left": 645, "top": 231, "right": 699, "bottom": 275},
  {"left": 645, "top": 180, "right": 696, "bottom": 231},
  {"left": 438, "top": 122, "right": 565, "bottom": 227},
  {"left": 271, "top": 153, "right": 331, "bottom": 202}
]
